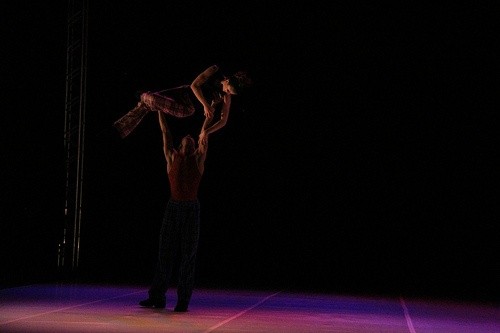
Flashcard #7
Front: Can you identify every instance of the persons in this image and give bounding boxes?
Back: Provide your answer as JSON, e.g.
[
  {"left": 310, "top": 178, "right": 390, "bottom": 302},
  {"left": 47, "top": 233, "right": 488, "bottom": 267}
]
[
  {"left": 111, "top": 64, "right": 252, "bottom": 140},
  {"left": 138, "top": 99, "right": 215, "bottom": 314}
]
